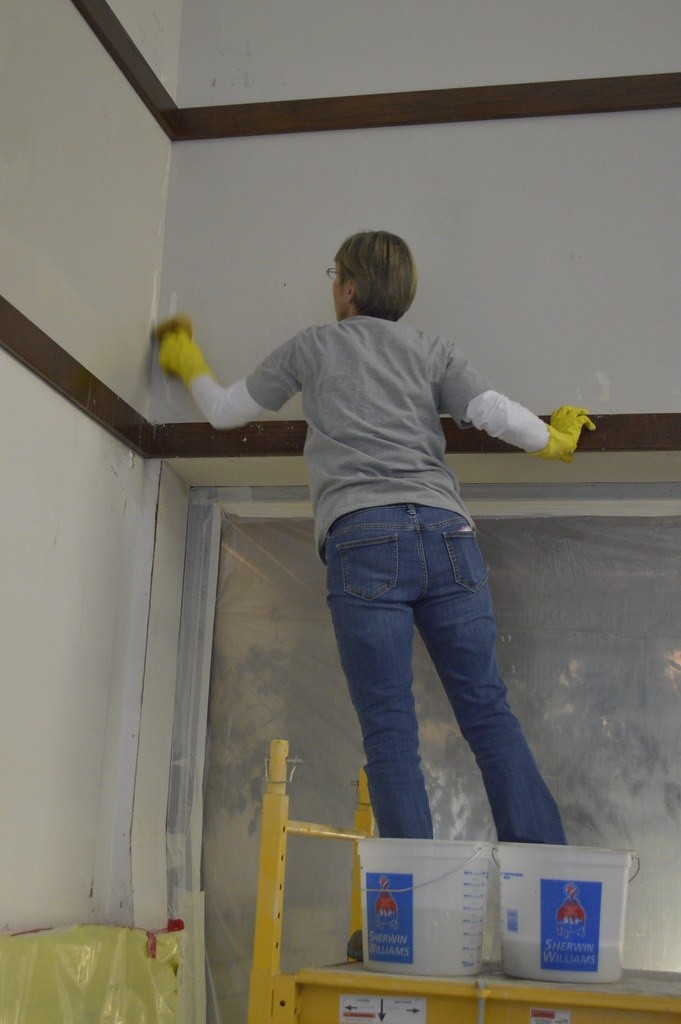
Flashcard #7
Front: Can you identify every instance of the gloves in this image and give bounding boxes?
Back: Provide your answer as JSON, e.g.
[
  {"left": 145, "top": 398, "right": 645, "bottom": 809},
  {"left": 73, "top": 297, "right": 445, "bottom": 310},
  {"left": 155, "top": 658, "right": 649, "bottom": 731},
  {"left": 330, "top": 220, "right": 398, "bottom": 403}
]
[
  {"left": 527, "top": 404, "right": 596, "bottom": 465},
  {"left": 158, "top": 316, "right": 219, "bottom": 395}
]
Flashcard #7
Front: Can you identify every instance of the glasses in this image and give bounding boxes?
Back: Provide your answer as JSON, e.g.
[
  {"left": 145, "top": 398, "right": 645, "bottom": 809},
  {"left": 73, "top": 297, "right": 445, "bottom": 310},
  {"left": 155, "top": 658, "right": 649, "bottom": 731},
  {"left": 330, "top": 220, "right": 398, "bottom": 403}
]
[{"left": 327, "top": 267, "right": 347, "bottom": 279}]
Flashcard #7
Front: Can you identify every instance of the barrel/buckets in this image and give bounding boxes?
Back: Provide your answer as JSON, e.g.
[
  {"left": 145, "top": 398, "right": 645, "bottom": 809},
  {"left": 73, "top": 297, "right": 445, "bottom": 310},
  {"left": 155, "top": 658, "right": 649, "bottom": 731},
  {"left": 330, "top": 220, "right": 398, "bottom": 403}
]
[
  {"left": 492, "top": 842, "right": 640, "bottom": 983},
  {"left": 355, "top": 838, "right": 492, "bottom": 976}
]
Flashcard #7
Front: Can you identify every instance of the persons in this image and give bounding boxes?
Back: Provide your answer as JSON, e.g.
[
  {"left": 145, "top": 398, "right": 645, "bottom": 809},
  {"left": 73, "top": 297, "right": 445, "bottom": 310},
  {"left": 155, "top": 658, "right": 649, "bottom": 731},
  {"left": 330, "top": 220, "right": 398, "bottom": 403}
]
[{"left": 156, "top": 232, "right": 597, "bottom": 962}]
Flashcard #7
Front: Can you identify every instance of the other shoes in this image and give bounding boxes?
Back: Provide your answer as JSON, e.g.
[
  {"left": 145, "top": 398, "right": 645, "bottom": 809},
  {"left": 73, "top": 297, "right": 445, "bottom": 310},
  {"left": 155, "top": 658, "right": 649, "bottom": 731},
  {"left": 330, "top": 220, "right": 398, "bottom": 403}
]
[{"left": 346, "top": 929, "right": 363, "bottom": 961}]
